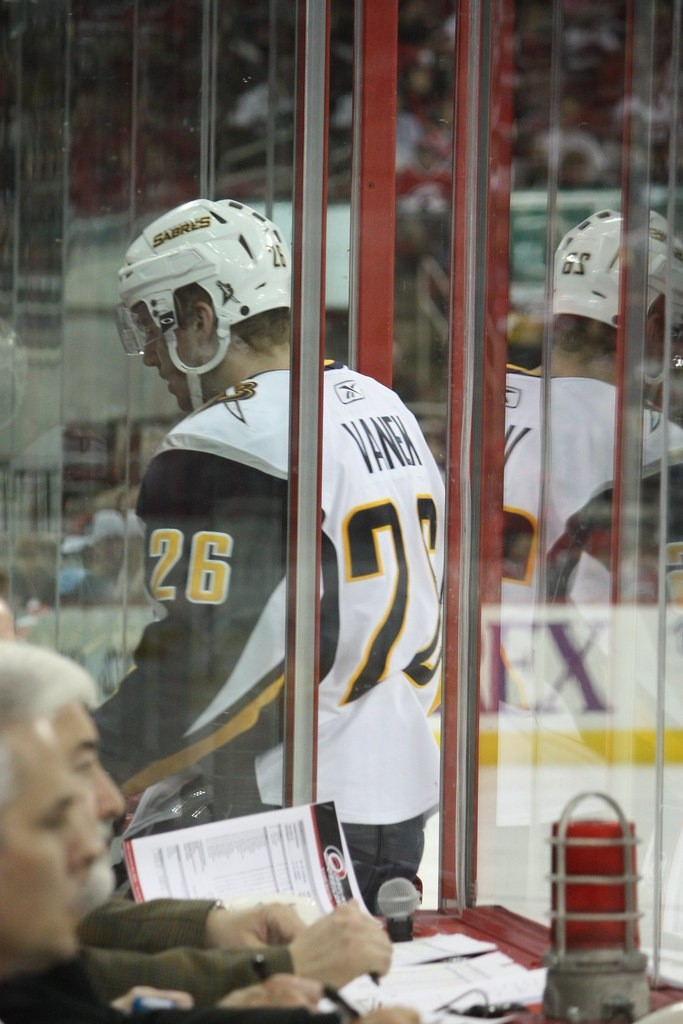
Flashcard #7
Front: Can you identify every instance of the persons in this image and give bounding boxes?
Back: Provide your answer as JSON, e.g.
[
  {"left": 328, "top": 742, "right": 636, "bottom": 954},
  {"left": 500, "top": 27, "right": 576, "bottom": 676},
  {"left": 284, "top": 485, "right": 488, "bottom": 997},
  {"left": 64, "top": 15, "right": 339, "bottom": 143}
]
[
  {"left": 465, "top": 199, "right": 683, "bottom": 931},
  {"left": 2, "top": 0, "right": 682, "bottom": 412},
  {"left": 1, "top": 630, "right": 398, "bottom": 1006},
  {"left": 0, "top": 640, "right": 419, "bottom": 1023},
  {"left": 75, "top": 194, "right": 452, "bottom": 952}
]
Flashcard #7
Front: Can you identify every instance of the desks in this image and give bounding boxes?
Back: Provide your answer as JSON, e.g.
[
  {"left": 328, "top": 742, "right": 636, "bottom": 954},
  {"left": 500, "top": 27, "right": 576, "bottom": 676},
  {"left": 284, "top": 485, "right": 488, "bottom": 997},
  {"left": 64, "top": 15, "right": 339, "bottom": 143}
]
[{"left": 379, "top": 905, "right": 679, "bottom": 1024}]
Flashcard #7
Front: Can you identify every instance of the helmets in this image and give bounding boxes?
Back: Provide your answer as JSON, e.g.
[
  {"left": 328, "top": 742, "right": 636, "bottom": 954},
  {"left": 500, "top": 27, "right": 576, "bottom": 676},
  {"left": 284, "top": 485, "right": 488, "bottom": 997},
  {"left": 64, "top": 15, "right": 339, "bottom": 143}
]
[
  {"left": 551, "top": 209, "right": 683, "bottom": 335},
  {"left": 115, "top": 197, "right": 299, "bottom": 373}
]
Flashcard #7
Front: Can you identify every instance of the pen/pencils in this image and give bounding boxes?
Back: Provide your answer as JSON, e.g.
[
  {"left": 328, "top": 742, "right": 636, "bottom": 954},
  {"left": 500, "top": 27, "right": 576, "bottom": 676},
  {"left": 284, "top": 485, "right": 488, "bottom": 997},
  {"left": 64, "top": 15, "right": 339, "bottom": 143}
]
[
  {"left": 369, "top": 973, "right": 379, "bottom": 986},
  {"left": 252, "top": 954, "right": 271, "bottom": 981},
  {"left": 323, "top": 987, "right": 359, "bottom": 1019}
]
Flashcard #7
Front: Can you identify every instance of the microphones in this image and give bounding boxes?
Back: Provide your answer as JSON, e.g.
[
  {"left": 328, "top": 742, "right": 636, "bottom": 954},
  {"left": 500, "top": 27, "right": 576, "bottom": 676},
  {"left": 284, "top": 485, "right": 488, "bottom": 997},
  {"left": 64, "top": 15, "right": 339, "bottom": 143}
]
[{"left": 377, "top": 877, "right": 421, "bottom": 942}]
